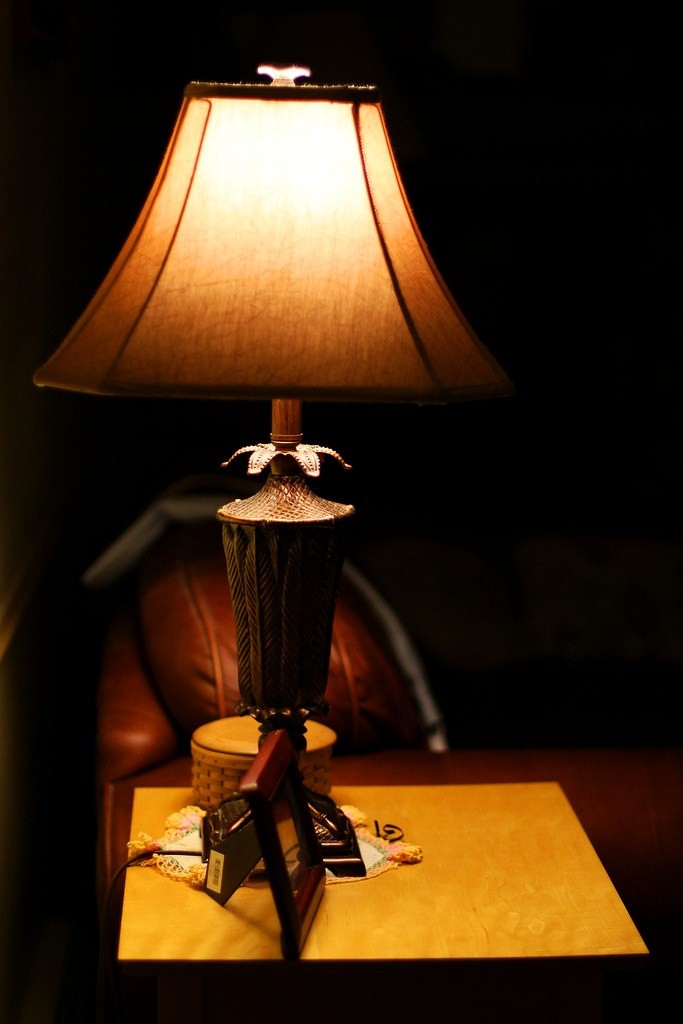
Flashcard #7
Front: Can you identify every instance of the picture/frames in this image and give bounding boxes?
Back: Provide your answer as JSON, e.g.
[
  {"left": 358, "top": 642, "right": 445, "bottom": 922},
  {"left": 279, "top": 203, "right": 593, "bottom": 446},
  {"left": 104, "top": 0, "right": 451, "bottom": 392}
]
[{"left": 239, "top": 730, "right": 325, "bottom": 958}]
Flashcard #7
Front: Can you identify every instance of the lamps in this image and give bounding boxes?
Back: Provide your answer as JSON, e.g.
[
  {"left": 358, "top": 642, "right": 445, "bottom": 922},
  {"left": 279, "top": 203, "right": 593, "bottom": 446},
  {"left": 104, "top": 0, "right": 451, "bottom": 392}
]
[{"left": 32, "top": 65, "right": 517, "bottom": 877}]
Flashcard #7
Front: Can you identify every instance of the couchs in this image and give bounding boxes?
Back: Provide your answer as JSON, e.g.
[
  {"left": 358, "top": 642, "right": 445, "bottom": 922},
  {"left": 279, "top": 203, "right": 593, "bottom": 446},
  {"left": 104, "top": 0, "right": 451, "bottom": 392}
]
[{"left": 95, "top": 534, "right": 683, "bottom": 1024}]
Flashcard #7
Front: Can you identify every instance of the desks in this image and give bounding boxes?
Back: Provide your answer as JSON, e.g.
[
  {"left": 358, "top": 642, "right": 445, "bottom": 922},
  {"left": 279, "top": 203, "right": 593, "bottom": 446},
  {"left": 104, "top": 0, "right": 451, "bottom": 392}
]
[{"left": 116, "top": 781, "right": 650, "bottom": 1024}]
